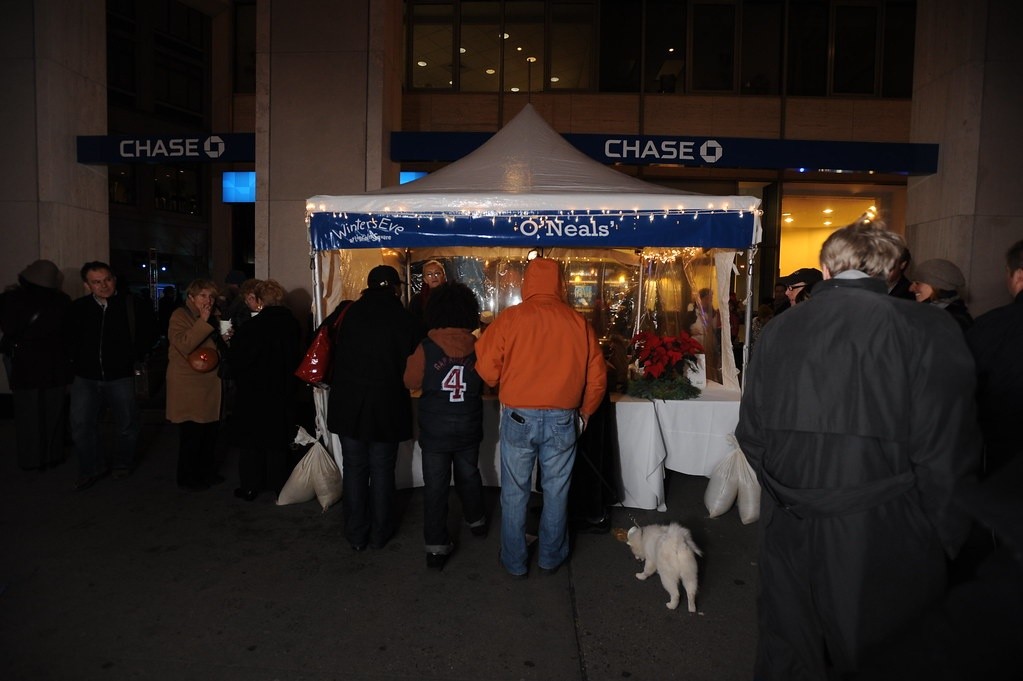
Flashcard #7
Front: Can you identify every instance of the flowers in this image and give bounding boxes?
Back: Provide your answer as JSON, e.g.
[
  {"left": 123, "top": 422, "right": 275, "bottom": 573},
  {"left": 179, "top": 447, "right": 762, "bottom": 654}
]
[{"left": 620, "top": 332, "right": 704, "bottom": 404}]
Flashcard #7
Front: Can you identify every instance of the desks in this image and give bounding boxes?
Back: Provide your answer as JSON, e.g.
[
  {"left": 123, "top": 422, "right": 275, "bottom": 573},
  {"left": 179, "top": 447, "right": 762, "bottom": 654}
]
[{"left": 332, "top": 380, "right": 742, "bottom": 513}]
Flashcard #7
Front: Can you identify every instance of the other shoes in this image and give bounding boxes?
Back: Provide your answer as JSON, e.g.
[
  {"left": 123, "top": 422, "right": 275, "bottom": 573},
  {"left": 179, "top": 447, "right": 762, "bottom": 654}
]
[
  {"left": 538, "top": 549, "right": 569, "bottom": 576},
  {"left": 425, "top": 535, "right": 458, "bottom": 568},
  {"left": 74, "top": 470, "right": 105, "bottom": 489},
  {"left": 110, "top": 467, "right": 128, "bottom": 479},
  {"left": 175, "top": 474, "right": 224, "bottom": 490},
  {"left": 471, "top": 526, "right": 486, "bottom": 539},
  {"left": 497, "top": 547, "right": 529, "bottom": 580}
]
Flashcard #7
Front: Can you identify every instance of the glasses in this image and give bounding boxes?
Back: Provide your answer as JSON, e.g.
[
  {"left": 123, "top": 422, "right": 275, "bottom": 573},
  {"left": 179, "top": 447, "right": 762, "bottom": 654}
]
[{"left": 788, "top": 285, "right": 803, "bottom": 291}]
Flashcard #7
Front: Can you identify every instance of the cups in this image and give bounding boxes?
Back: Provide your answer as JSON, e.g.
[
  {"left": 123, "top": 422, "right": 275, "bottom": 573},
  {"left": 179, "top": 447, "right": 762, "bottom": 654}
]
[{"left": 220, "top": 320, "right": 232, "bottom": 335}]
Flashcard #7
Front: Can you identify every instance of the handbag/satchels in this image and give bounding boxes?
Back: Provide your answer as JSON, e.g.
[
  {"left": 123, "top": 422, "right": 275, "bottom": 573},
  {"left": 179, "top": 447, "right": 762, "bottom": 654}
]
[
  {"left": 295, "top": 303, "right": 355, "bottom": 385},
  {"left": 187, "top": 348, "right": 220, "bottom": 373},
  {"left": 134, "top": 365, "right": 164, "bottom": 400}
]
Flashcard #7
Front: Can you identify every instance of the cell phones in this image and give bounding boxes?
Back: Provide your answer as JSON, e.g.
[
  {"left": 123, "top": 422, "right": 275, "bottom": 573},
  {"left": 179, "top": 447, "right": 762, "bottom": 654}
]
[{"left": 511, "top": 411, "right": 525, "bottom": 424}]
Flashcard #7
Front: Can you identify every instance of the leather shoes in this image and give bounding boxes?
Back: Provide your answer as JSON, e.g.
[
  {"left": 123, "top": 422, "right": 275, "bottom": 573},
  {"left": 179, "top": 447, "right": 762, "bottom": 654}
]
[{"left": 244, "top": 486, "right": 257, "bottom": 497}]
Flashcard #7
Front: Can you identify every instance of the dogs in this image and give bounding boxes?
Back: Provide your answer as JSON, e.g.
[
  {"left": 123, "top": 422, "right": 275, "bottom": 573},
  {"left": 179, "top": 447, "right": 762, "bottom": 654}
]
[{"left": 625, "top": 520, "right": 705, "bottom": 613}]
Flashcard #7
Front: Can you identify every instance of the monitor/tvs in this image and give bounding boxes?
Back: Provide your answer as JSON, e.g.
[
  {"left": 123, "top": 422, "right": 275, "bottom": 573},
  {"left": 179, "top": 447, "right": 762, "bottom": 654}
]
[
  {"left": 399, "top": 171, "right": 430, "bottom": 186},
  {"left": 220, "top": 171, "right": 255, "bottom": 205}
]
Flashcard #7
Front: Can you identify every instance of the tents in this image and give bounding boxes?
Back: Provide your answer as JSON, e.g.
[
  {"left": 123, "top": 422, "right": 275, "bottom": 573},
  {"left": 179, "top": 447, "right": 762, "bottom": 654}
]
[{"left": 302, "top": 106, "right": 761, "bottom": 401}]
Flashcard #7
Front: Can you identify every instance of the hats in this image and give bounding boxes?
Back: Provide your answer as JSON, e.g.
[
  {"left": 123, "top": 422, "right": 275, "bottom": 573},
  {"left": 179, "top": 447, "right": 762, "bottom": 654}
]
[
  {"left": 368, "top": 264, "right": 405, "bottom": 288},
  {"left": 911, "top": 259, "right": 965, "bottom": 290},
  {"left": 780, "top": 269, "right": 820, "bottom": 285},
  {"left": 17, "top": 258, "right": 59, "bottom": 288}
]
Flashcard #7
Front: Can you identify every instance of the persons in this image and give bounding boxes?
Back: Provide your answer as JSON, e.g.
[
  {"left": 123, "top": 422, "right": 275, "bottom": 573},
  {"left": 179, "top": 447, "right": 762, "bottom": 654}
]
[
  {"left": 734, "top": 220, "right": 979, "bottom": 681},
  {"left": 684, "top": 268, "right": 823, "bottom": 375},
  {"left": 0, "top": 260, "right": 74, "bottom": 471},
  {"left": 404, "top": 281, "right": 489, "bottom": 574},
  {"left": 409, "top": 260, "right": 448, "bottom": 347},
  {"left": 886, "top": 242, "right": 1023, "bottom": 592},
  {"left": 470, "top": 258, "right": 620, "bottom": 577},
  {"left": 308, "top": 265, "right": 413, "bottom": 551},
  {"left": 160, "top": 280, "right": 315, "bottom": 502},
  {"left": 66, "top": 262, "right": 153, "bottom": 491}
]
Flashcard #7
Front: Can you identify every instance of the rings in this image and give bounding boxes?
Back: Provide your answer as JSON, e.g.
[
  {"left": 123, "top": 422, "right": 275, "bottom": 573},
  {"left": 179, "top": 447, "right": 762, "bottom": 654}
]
[{"left": 142, "top": 370, "right": 145, "bottom": 372}]
